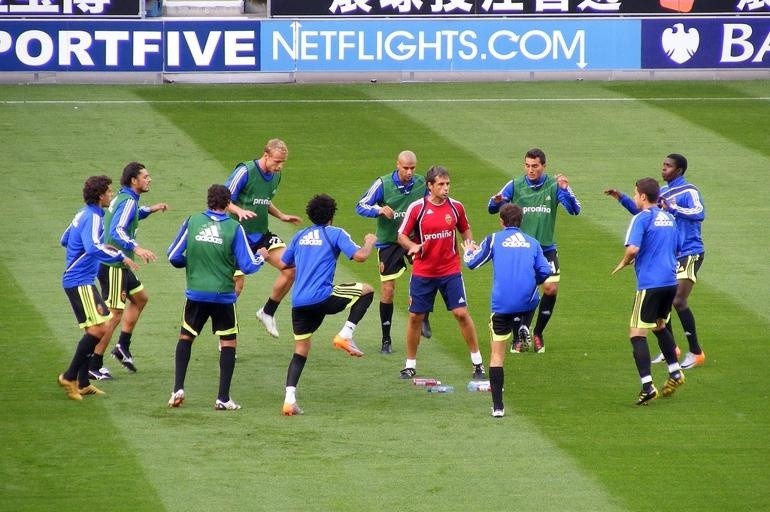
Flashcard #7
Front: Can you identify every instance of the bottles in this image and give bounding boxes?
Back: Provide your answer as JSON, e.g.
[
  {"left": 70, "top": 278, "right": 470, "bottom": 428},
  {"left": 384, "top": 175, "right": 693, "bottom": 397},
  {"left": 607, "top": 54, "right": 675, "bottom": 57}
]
[
  {"left": 465, "top": 379, "right": 505, "bottom": 392},
  {"left": 427, "top": 385, "right": 453, "bottom": 394},
  {"left": 414, "top": 378, "right": 442, "bottom": 386}
]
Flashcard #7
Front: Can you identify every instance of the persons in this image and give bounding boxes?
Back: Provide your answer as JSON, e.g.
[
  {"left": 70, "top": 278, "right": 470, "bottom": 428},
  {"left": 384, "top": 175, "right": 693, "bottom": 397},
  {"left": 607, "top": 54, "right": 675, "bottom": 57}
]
[
  {"left": 610, "top": 175, "right": 689, "bottom": 407},
  {"left": 88, "top": 160, "right": 169, "bottom": 385},
  {"left": 487, "top": 147, "right": 581, "bottom": 354},
  {"left": 354, "top": 149, "right": 434, "bottom": 356},
  {"left": 394, "top": 164, "right": 488, "bottom": 384},
  {"left": 222, "top": 137, "right": 296, "bottom": 341},
  {"left": 602, "top": 154, "right": 706, "bottom": 371},
  {"left": 457, "top": 205, "right": 553, "bottom": 423},
  {"left": 57, "top": 174, "right": 131, "bottom": 404},
  {"left": 277, "top": 193, "right": 378, "bottom": 415},
  {"left": 164, "top": 184, "right": 268, "bottom": 415}
]
